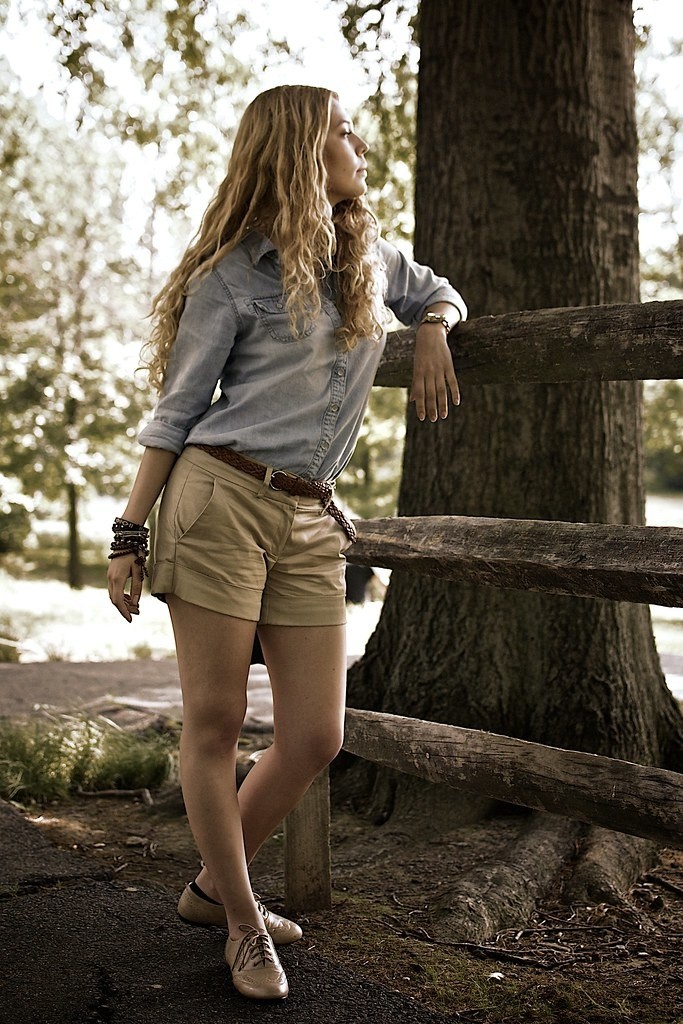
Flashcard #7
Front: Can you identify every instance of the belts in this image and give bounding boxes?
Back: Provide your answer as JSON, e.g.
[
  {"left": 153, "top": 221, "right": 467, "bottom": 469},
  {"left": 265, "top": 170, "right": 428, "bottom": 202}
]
[{"left": 195, "top": 442, "right": 358, "bottom": 545}]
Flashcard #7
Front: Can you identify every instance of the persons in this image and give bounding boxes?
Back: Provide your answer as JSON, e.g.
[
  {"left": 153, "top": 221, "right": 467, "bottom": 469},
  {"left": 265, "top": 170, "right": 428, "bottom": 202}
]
[{"left": 106, "top": 83, "right": 466, "bottom": 998}]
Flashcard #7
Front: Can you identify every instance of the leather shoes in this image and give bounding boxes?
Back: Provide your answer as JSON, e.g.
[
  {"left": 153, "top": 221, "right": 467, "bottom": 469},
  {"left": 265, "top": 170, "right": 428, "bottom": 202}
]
[
  {"left": 178, "top": 880, "right": 304, "bottom": 946},
  {"left": 224, "top": 923, "right": 289, "bottom": 1001}
]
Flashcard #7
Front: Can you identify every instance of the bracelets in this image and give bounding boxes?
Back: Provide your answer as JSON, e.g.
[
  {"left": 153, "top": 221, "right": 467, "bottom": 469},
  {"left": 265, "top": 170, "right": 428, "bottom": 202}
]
[
  {"left": 418, "top": 313, "right": 450, "bottom": 334},
  {"left": 108, "top": 517, "right": 149, "bottom": 582}
]
